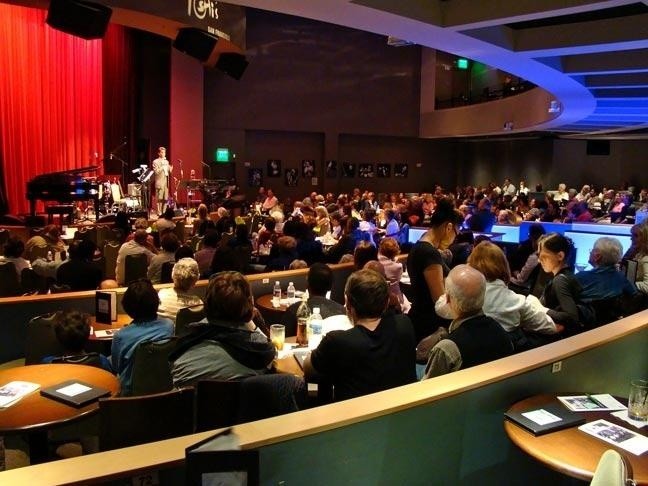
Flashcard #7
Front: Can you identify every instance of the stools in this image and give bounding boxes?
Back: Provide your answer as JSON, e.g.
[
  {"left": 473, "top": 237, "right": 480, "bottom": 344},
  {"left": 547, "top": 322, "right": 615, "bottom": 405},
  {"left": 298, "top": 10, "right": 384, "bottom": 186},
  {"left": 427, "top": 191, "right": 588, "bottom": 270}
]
[{"left": 47, "top": 204, "right": 74, "bottom": 227}]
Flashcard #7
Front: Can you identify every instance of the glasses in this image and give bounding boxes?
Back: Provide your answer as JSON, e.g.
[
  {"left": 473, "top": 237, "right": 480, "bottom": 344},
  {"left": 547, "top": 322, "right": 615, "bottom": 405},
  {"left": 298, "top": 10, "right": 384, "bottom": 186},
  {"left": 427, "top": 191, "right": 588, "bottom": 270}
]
[
  {"left": 588, "top": 248, "right": 597, "bottom": 258},
  {"left": 452, "top": 225, "right": 459, "bottom": 237}
]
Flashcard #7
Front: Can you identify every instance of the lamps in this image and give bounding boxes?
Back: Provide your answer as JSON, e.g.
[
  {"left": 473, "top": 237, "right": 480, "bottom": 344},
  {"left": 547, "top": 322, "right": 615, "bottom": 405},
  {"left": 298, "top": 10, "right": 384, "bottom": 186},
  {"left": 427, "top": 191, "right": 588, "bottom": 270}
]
[
  {"left": 44, "top": 1, "right": 111, "bottom": 39},
  {"left": 173, "top": 27, "right": 217, "bottom": 64},
  {"left": 218, "top": 54, "right": 248, "bottom": 78}
]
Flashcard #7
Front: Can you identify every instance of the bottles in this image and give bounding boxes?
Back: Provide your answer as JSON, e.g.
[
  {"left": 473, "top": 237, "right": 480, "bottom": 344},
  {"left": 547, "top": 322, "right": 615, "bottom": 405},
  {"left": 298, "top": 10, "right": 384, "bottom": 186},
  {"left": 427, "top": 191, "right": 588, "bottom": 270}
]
[
  {"left": 272, "top": 281, "right": 281, "bottom": 307},
  {"left": 286, "top": 281, "right": 296, "bottom": 307},
  {"left": 308, "top": 307, "right": 323, "bottom": 351},
  {"left": 296, "top": 294, "right": 310, "bottom": 346},
  {"left": 47, "top": 250, "right": 52, "bottom": 260}
]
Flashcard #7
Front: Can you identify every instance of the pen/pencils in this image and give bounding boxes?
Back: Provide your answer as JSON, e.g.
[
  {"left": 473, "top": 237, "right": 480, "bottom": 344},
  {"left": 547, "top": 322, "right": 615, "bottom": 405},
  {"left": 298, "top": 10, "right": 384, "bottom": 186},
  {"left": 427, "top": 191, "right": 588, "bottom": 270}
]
[{"left": 586, "top": 393, "right": 603, "bottom": 407}]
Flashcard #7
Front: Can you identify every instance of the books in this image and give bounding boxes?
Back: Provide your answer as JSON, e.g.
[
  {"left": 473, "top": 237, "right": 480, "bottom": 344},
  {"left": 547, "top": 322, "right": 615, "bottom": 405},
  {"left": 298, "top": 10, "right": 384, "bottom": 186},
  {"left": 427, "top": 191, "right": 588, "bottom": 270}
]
[
  {"left": 621, "top": 256, "right": 638, "bottom": 284},
  {"left": 504, "top": 402, "right": 587, "bottom": 437},
  {"left": 39, "top": 380, "right": 110, "bottom": 408},
  {"left": 95, "top": 290, "right": 118, "bottom": 324},
  {"left": 186, "top": 428, "right": 260, "bottom": 485}
]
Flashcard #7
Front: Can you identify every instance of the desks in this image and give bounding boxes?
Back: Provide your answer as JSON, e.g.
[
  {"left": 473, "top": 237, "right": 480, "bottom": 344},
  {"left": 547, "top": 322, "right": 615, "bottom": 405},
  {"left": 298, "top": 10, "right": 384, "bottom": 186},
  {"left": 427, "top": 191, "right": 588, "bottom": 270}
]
[
  {"left": 504, "top": 390, "right": 648, "bottom": 485},
  {"left": 89, "top": 306, "right": 134, "bottom": 348},
  {"left": 268, "top": 329, "right": 334, "bottom": 389},
  {"left": 256, "top": 290, "right": 311, "bottom": 325},
  {"left": 1, "top": 363, "right": 122, "bottom": 464},
  {"left": 399, "top": 269, "right": 414, "bottom": 288}
]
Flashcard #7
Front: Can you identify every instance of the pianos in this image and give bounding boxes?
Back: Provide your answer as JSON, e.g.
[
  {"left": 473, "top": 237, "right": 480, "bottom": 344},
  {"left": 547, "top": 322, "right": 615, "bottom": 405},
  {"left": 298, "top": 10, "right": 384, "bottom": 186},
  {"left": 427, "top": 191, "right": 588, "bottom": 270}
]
[
  {"left": 181, "top": 178, "right": 228, "bottom": 208},
  {"left": 25, "top": 165, "right": 100, "bottom": 222}
]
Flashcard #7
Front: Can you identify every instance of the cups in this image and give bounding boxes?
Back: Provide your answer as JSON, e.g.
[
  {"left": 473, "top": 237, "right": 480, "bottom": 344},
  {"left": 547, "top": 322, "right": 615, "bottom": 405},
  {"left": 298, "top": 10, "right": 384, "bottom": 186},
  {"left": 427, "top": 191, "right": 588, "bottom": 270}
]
[
  {"left": 270, "top": 324, "right": 286, "bottom": 351},
  {"left": 627, "top": 379, "right": 648, "bottom": 422}
]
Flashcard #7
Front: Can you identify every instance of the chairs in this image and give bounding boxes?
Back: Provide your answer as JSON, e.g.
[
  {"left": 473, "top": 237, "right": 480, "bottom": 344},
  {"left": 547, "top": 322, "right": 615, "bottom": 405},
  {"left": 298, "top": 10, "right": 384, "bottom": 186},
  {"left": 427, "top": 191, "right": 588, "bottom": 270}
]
[
  {"left": 100, "top": 385, "right": 197, "bottom": 450},
  {"left": 172, "top": 303, "right": 206, "bottom": 335},
  {"left": 202, "top": 374, "right": 301, "bottom": 430},
  {"left": 130, "top": 336, "right": 173, "bottom": 395}
]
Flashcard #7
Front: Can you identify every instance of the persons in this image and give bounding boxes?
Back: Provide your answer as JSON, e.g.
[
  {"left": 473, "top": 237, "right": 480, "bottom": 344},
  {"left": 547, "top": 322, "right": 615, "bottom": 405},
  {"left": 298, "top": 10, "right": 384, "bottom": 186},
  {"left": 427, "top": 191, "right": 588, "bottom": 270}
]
[
  {"left": 538, "top": 233, "right": 581, "bottom": 342},
  {"left": 574, "top": 236, "right": 648, "bottom": 330},
  {"left": 510, "top": 224, "right": 547, "bottom": 287},
  {"left": 434, "top": 240, "right": 558, "bottom": 352},
  {"left": 153, "top": 258, "right": 209, "bottom": 327},
  {"left": 417, "top": 178, "right": 648, "bottom": 233},
  {"left": 285, "top": 263, "right": 346, "bottom": 336},
  {"left": 302, "top": 274, "right": 417, "bottom": 405},
  {"left": 621, "top": 223, "right": 648, "bottom": 292},
  {"left": 448, "top": 234, "right": 471, "bottom": 269},
  {"left": 172, "top": 186, "right": 415, "bottom": 272},
  {"left": 40, "top": 308, "right": 112, "bottom": 373},
  {"left": 407, "top": 198, "right": 465, "bottom": 367},
  {"left": 422, "top": 264, "right": 515, "bottom": 379},
  {"left": 111, "top": 280, "right": 174, "bottom": 396},
  {"left": 509, "top": 251, "right": 549, "bottom": 298},
  {"left": 146, "top": 270, "right": 276, "bottom": 389},
  {"left": 473, "top": 234, "right": 491, "bottom": 248},
  {"left": 1, "top": 146, "right": 173, "bottom": 288}
]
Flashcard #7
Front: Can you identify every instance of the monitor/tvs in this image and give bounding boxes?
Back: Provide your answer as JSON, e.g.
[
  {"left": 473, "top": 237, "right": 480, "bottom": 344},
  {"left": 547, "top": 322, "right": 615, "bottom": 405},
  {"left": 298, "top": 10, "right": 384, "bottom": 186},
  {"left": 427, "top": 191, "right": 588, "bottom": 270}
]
[{"left": 213, "top": 146, "right": 232, "bottom": 164}]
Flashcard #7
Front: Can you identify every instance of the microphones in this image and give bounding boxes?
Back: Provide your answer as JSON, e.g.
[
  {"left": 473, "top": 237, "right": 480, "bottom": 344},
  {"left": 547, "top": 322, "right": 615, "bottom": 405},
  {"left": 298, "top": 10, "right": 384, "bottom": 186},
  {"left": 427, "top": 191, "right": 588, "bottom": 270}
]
[
  {"left": 176, "top": 158, "right": 183, "bottom": 165},
  {"left": 198, "top": 161, "right": 211, "bottom": 168}
]
